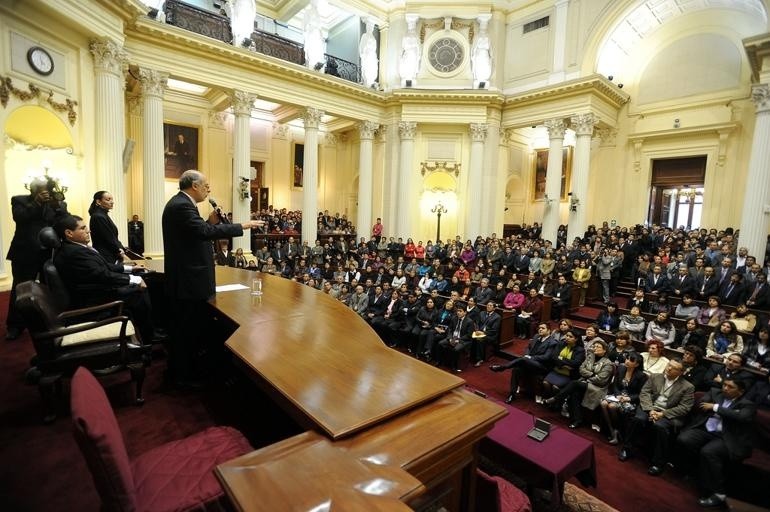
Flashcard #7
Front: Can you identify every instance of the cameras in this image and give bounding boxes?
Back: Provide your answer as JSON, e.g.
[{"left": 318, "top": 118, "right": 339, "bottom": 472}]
[{"left": 47, "top": 180, "right": 66, "bottom": 201}]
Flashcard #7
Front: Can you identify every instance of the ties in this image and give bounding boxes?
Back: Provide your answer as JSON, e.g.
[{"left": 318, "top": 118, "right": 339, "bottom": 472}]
[
  {"left": 453, "top": 319, "right": 461, "bottom": 340},
  {"left": 706, "top": 399, "right": 733, "bottom": 432}
]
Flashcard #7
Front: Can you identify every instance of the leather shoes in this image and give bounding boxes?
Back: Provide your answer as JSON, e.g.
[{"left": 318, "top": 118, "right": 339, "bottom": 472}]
[
  {"left": 618, "top": 450, "right": 627, "bottom": 462},
  {"left": 4, "top": 327, "right": 20, "bottom": 340},
  {"left": 504, "top": 395, "right": 514, "bottom": 404},
  {"left": 390, "top": 344, "right": 432, "bottom": 362},
  {"left": 518, "top": 333, "right": 527, "bottom": 339},
  {"left": 474, "top": 359, "right": 484, "bottom": 367},
  {"left": 488, "top": 364, "right": 507, "bottom": 372},
  {"left": 143, "top": 332, "right": 171, "bottom": 344},
  {"left": 648, "top": 465, "right": 662, "bottom": 475},
  {"left": 541, "top": 396, "right": 555, "bottom": 407},
  {"left": 454, "top": 369, "right": 463, "bottom": 372},
  {"left": 697, "top": 493, "right": 727, "bottom": 507},
  {"left": 569, "top": 420, "right": 582, "bottom": 428}
]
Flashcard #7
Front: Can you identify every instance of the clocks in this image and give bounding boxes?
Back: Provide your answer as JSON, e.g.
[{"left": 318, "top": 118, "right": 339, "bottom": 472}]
[{"left": 26, "top": 44, "right": 55, "bottom": 77}]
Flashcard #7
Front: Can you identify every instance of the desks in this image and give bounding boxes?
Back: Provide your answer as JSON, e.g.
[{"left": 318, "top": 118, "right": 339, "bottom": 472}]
[{"left": 464, "top": 384, "right": 598, "bottom": 508}]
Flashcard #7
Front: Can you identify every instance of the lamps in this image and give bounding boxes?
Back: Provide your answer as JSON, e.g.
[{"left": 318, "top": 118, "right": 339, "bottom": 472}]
[
  {"left": 419, "top": 191, "right": 462, "bottom": 242},
  {"left": 478, "top": 82, "right": 486, "bottom": 89},
  {"left": 608, "top": 73, "right": 625, "bottom": 90},
  {"left": 146, "top": 6, "right": 326, "bottom": 72},
  {"left": 405, "top": 80, "right": 413, "bottom": 88},
  {"left": 20, "top": 158, "right": 73, "bottom": 196}
]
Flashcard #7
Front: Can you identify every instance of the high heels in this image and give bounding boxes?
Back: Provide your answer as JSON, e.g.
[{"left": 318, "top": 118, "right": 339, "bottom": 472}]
[{"left": 608, "top": 429, "right": 623, "bottom": 444}]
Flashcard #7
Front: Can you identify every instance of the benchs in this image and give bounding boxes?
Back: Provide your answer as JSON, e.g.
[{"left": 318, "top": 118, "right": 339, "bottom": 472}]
[
  {"left": 224, "top": 210, "right": 547, "bottom": 367},
  {"left": 528, "top": 227, "right": 770, "bottom": 490}
]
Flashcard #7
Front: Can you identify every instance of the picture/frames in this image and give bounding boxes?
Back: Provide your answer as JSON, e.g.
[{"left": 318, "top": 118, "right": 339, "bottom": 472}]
[
  {"left": 289, "top": 139, "right": 321, "bottom": 192},
  {"left": 532, "top": 144, "right": 574, "bottom": 204},
  {"left": 162, "top": 117, "right": 204, "bottom": 183}
]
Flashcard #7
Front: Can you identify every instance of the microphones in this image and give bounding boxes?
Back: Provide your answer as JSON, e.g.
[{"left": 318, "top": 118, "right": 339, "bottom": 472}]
[{"left": 207, "top": 197, "right": 229, "bottom": 223}]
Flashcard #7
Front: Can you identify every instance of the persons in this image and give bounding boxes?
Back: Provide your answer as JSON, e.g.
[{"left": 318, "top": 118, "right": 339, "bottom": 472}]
[
  {"left": 4, "top": 180, "right": 60, "bottom": 340},
  {"left": 170, "top": 133, "right": 192, "bottom": 171},
  {"left": 129, "top": 214, "right": 144, "bottom": 257},
  {"left": 162, "top": 168, "right": 264, "bottom": 381},
  {"left": 58, "top": 215, "right": 166, "bottom": 342},
  {"left": 89, "top": 191, "right": 125, "bottom": 265}
]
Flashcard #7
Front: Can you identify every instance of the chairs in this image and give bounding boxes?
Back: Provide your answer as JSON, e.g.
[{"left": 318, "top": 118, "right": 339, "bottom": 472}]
[
  {"left": 64, "top": 363, "right": 259, "bottom": 511},
  {"left": 472, "top": 465, "right": 536, "bottom": 512},
  {"left": 11, "top": 277, "right": 146, "bottom": 410},
  {"left": 36, "top": 224, "right": 158, "bottom": 375}
]
